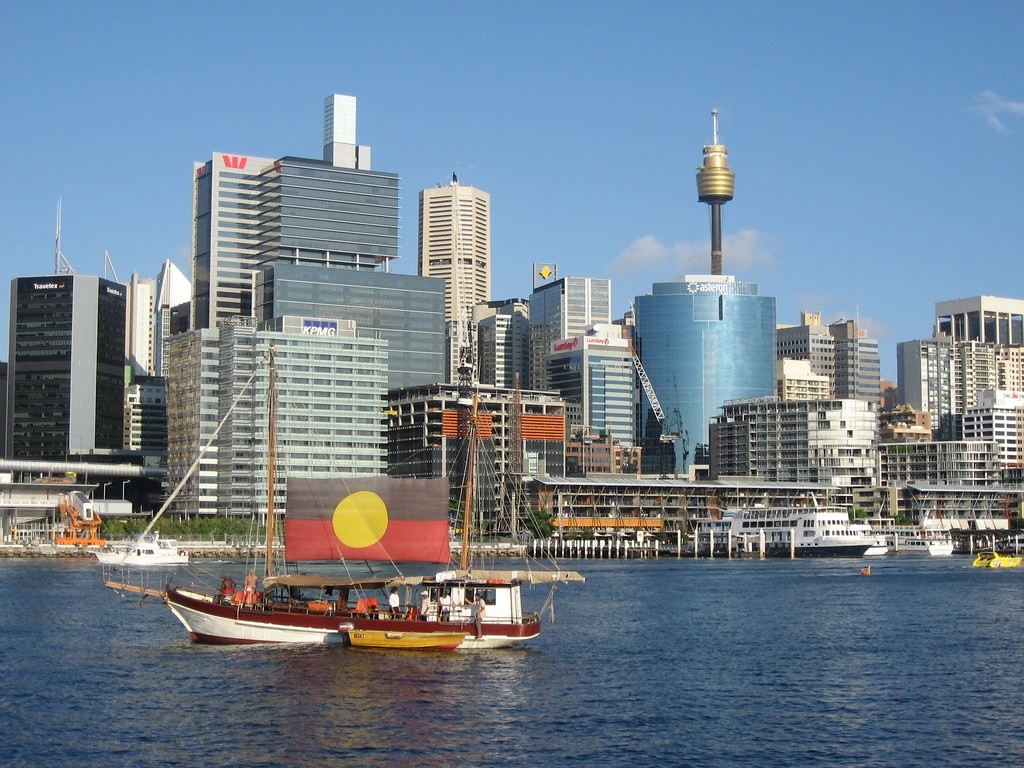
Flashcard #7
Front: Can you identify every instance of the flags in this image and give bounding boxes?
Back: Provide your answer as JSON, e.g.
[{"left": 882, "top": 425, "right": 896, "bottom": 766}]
[{"left": 285, "top": 477, "right": 450, "bottom": 564}]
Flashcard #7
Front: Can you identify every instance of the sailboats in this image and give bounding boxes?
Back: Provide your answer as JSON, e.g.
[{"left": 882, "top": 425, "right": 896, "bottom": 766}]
[{"left": 103, "top": 340, "right": 585, "bottom": 647}]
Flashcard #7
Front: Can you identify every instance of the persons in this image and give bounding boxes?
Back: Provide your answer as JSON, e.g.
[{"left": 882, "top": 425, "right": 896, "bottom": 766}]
[
  {"left": 221, "top": 574, "right": 235, "bottom": 594},
  {"left": 1011, "top": 553, "right": 1014, "bottom": 558},
  {"left": 439, "top": 591, "right": 450, "bottom": 623},
  {"left": 464, "top": 593, "right": 486, "bottom": 639},
  {"left": 986, "top": 554, "right": 991, "bottom": 560},
  {"left": 389, "top": 586, "right": 399, "bottom": 619},
  {"left": 243, "top": 568, "right": 258, "bottom": 588},
  {"left": 420, "top": 591, "right": 430, "bottom": 621}
]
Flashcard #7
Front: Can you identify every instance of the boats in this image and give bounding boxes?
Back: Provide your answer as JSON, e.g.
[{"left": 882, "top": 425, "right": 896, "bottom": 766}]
[
  {"left": 973, "top": 551, "right": 1022, "bottom": 568},
  {"left": 94, "top": 531, "right": 190, "bottom": 567},
  {"left": 884, "top": 528, "right": 955, "bottom": 557},
  {"left": 694, "top": 507, "right": 878, "bottom": 558},
  {"left": 335, "top": 622, "right": 470, "bottom": 653}
]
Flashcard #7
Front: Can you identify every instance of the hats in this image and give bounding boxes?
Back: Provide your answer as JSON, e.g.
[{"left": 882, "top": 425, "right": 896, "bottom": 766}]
[{"left": 421, "top": 591, "right": 428, "bottom": 596}]
